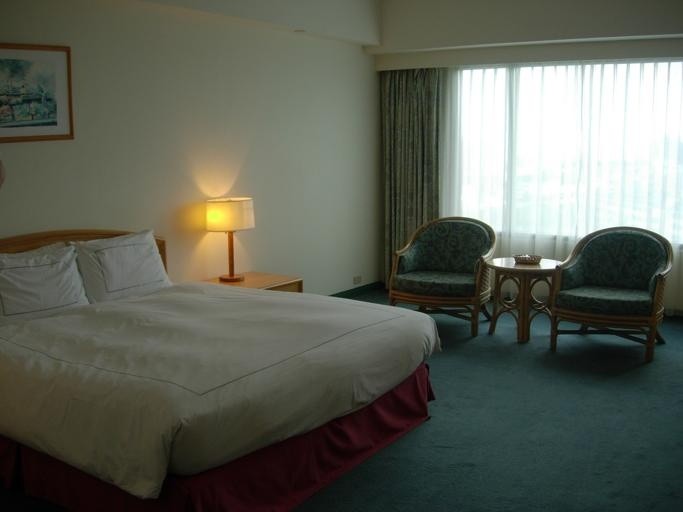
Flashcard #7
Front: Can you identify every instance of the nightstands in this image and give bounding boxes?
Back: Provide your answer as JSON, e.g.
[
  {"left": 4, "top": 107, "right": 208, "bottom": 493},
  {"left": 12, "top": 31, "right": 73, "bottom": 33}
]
[{"left": 200, "top": 272, "right": 304, "bottom": 293}]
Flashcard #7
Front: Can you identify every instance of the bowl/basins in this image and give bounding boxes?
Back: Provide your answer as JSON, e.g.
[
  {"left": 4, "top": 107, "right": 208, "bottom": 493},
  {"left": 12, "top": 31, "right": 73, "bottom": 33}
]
[{"left": 512, "top": 255, "right": 544, "bottom": 264}]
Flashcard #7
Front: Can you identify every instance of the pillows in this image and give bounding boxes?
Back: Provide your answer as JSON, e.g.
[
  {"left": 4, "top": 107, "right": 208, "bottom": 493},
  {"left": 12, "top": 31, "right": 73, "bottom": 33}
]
[
  {"left": 0, "top": 242, "right": 90, "bottom": 321},
  {"left": 67, "top": 231, "right": 172, "bottom": 304}
]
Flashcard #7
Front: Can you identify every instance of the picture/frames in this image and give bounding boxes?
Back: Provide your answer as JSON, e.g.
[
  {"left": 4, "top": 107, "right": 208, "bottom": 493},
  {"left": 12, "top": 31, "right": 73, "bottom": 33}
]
[{"left": 0, "top": 43, "right": 75, "bottom": 144}]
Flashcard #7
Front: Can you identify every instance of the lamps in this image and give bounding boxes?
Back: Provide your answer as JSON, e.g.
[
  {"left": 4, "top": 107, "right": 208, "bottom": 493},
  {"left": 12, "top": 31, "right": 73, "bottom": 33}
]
[{"left": 206, "top": 197, "right": 255, "bottom": 282}]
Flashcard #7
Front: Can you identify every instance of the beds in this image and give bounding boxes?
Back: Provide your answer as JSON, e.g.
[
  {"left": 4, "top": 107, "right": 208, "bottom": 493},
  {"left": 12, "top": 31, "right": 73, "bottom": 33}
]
[{"left": 0, "top": 230, "right": 442, "bottom": 512}]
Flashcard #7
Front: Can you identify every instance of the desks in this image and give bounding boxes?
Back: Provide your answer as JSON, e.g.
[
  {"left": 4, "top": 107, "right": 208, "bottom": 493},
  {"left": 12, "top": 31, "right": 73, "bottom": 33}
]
[{"left": 485, "top": 256, "right": 563, "bottom": 345}]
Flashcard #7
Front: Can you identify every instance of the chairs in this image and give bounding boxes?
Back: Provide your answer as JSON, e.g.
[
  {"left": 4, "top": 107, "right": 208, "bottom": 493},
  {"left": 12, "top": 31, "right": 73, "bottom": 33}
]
[
  {"left": 550, "top": 227, "right": 673, "bottom": 363},
  {"left": 389, "top": 216, "right": 495, "bottom": 338}
]
